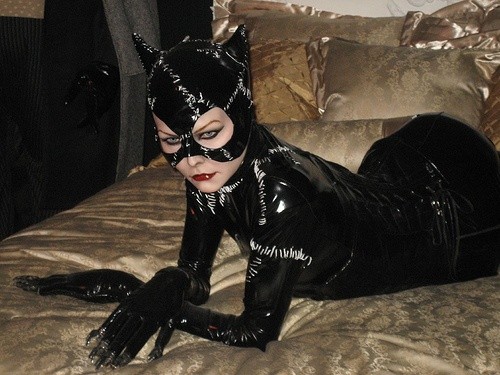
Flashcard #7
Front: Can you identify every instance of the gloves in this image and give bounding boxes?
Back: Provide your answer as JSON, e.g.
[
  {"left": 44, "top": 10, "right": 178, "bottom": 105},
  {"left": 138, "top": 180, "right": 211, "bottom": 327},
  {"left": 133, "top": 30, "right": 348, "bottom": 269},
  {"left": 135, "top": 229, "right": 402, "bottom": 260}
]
[
  {"left": 86, "top": 267, "right": 191, "bottom": 368},
  {"left": 15, "top": 268, "right": 131, "bottom": 306}
]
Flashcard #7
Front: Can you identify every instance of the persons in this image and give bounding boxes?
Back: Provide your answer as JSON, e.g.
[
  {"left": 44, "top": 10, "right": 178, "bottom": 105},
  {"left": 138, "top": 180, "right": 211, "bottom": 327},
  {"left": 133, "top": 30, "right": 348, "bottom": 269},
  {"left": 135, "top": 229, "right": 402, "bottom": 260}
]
[{"left": 13, "top": 25, "right": 500, "bottom": 370}]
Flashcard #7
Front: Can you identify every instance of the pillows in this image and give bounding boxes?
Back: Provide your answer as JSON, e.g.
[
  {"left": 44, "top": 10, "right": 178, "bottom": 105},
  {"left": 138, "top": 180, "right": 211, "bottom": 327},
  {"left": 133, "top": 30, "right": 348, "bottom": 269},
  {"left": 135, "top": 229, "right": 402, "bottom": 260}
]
[
  {"left": 213, "top": 0, "right": 403, "bottom": 46},
  {"left": 429, "top": 0, "right": 500, "bottom": 38},
  {"left": 256, "top": 113, "right": 418, "bottom": 176},
  {"left": 246, "top": 39, "right": 320, "bottom": 127},
  {"left": 394, "top": 8, "right": 482, "bottom": 52},
  {"left": 307, "top": 29, "right": 500, "bottom": 129}
]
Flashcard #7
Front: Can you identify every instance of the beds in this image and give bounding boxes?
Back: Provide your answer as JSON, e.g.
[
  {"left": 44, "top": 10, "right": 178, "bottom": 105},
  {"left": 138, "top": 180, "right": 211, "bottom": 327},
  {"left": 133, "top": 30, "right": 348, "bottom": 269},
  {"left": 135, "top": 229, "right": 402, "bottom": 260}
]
[{"left": 0, "top": 0, "right": 500, "bottom": 375}]
